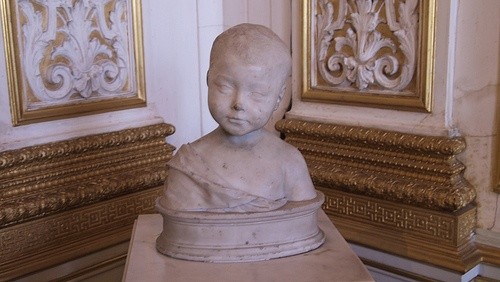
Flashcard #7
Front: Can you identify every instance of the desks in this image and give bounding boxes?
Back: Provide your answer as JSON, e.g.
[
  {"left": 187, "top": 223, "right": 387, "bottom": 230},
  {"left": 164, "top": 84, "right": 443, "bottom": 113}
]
[{"left": 122, "top": 202, "right": 373, "bottom": 282}]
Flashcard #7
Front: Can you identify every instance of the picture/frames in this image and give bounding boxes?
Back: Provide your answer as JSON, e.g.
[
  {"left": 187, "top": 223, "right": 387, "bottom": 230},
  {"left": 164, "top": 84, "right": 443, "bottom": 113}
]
[
  {"left": 299, "top": 0, "right": 438, "bottom": 114},
  {"left": 1, "top": 0, "right": 147, "bottom": 128}
]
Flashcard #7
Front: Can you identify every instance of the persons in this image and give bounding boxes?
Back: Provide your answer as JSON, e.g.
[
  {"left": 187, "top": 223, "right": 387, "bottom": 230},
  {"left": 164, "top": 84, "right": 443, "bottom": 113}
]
[{"left": 159, "top": 23, "right": 317, "bottom": 213}]
